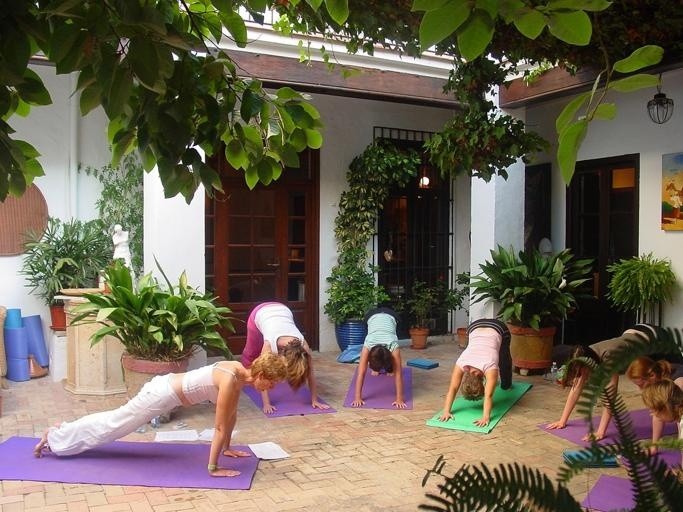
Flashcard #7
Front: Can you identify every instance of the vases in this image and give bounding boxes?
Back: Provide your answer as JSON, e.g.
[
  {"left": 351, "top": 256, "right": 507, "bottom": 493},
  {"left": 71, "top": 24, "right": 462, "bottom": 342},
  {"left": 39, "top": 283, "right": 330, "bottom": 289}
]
[{"left": 506, "top": 322, "right": 556, "bottom": 376}]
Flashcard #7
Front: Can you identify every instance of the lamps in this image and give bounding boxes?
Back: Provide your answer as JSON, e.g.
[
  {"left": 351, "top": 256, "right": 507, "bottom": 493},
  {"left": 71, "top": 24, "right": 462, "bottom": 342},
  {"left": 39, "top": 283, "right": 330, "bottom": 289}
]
[{"left": 647, "top": 72, "right": 674, "bottom": 124}]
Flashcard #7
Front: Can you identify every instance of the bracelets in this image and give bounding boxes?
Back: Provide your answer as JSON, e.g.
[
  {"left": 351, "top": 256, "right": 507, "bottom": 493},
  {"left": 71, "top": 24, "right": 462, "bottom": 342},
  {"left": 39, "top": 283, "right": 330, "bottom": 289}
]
[{"left": 208, "top": 465, "right": 217, "bottom": 471}]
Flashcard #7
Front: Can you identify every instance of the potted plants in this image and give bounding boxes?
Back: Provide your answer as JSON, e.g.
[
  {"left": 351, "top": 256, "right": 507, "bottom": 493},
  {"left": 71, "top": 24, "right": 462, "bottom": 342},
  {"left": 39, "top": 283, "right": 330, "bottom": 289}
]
[
  {"left": 324, "top": 136, "right": 421, "bottom": 352},
  {"left": 422, "top": 57, "right": 550, "bottom": 182},
  {"left": 392, "top": 279, "right": 444, "bottom": 347},
  {"left": 445, "top": 272, "right": 470, "bottom": 347},
  {"left": 69, "top": 253, "right": 246, "bottom": 401},
  {"left": 18, "top": 215, "right": 112, "bottom": 328}
]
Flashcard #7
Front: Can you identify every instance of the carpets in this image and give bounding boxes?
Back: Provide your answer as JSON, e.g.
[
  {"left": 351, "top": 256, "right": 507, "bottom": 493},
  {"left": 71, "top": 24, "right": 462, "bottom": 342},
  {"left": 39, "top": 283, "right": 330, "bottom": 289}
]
[
  {"left": 3, "top": 308, "right": 49, "bottom": 382},
  {"left": 425, "top": 377, "right": 533, "bottom": 434},
  {"left": 343, "top": 366, "right": 413, "bottom": 410},
  {"left": 243, "top": 381, "right": 338, "bottom": 418},
  {"left": 537, "top": 409, "right": 682, "bottom": 511},
  {"left": 0, "top": 436, "right": 260, "bottom": 490}
]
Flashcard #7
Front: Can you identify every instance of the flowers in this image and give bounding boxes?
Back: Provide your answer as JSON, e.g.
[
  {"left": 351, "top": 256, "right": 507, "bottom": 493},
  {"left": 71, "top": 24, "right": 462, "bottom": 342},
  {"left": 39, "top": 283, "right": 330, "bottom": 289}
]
[{"left": 464, "top": 243, "right": 595, "bottom": 331}]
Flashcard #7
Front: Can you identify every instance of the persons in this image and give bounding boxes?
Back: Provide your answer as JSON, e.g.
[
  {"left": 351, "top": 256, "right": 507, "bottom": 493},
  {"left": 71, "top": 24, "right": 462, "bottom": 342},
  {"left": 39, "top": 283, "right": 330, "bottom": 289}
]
[
  {"left": 35, "top": 350, "right": 288, "bottom": 478},
  {"left": 642, "top": 378, "right": 683, "bottom": 446},
  {"left": 626, "top": 356, "right": 683, "bottom": 457},
  {"left": 436, "top": 319, "right": 513, "bottom": 428},
  {"left": 546, "top": 322, "right": 683, "bottom": 442},
  {"left": 351, "top": 307, "right": 408, "bottom": 409},
  {"left": 241, "top": 302, "right": 330, "bottom": 414}
]
